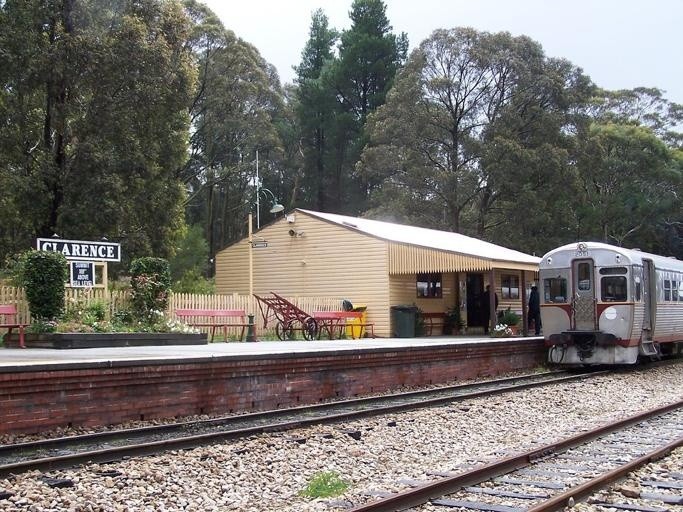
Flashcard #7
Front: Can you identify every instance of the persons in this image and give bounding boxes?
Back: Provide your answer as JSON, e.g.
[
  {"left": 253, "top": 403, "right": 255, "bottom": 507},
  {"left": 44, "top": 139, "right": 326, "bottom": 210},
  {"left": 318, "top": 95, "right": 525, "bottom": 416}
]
[
  {"left": 528, "top": 287, "right": 541, "bottom": 336},
  {"left": 479, "top": 284, "right": 499, "bottom": 335}
]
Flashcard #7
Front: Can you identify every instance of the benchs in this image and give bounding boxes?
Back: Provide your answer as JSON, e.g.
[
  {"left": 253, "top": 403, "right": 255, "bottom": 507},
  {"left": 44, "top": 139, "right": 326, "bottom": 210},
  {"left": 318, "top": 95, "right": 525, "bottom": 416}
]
[
  {"left": 414, "top": 310, "right": 452, "bottom": 336},
  {"left": 173, "top": 308, "right": 252, "bottom": 342},
  {"left": 312, "top": 311, "right": 374, "bottom": 338},
  {"left": 0, "top": 305, "right": 28, "bottom": 348}
]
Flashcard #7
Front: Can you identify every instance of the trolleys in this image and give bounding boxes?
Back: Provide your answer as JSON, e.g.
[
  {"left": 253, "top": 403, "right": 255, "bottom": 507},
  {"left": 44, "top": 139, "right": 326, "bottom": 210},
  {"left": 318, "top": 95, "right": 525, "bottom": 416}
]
[{"left": 252, "top": 292, "right": 317, "bottom": 341}]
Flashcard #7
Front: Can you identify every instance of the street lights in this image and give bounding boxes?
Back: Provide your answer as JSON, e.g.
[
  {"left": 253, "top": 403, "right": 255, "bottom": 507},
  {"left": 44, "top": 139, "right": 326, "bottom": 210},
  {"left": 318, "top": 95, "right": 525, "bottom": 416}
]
[{"left": 245, "top": 189, "right": 284, "bottom": 342}]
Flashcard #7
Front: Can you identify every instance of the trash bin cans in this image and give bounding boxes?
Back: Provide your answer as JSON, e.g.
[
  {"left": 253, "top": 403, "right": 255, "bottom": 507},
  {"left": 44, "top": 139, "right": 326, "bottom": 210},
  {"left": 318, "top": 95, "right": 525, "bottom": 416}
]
[
  {"left": 345, "top": 305, "right": 368, "bottom": 338},
  {"left": 391, "top": 305, "right": 417, "bottom": 338}
]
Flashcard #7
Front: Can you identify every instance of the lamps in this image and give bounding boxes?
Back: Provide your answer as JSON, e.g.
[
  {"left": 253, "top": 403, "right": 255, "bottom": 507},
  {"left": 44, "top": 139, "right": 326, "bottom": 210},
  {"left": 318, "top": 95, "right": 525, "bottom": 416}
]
[{"left": 258, "top": 187, "right": 284, "bottom": 215}]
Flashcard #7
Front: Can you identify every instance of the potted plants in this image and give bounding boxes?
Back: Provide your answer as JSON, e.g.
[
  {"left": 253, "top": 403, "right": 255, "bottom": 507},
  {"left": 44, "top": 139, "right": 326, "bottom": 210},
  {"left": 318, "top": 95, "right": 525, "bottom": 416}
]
[{"left": 500, "top": 311, "right": 519, "bottom": 336}]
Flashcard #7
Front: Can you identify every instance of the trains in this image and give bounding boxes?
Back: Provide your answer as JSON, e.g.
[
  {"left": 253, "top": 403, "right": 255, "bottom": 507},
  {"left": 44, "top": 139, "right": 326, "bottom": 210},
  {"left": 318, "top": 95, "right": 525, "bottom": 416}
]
[{"left": 539, "top": 242, "right": 683, "bottom": 364}]
